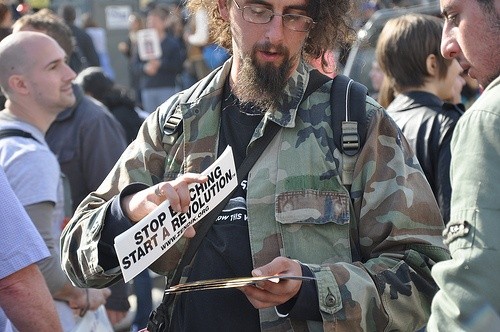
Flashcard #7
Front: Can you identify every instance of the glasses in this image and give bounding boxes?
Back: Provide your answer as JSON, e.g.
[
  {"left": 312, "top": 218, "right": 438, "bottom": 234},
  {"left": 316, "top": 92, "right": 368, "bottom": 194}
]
[{"left": 233, "top": 0, "right": 318, "bottom": 32}]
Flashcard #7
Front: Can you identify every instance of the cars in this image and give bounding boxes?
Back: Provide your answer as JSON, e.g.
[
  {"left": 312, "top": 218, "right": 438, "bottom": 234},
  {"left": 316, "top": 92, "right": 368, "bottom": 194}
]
[{"left": 342, "top": 2, "right": 447, "bottom": 103}]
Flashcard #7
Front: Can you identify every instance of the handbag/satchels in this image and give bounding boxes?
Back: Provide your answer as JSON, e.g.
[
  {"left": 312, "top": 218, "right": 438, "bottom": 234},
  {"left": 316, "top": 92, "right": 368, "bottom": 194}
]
[{"left": 138, "top": 309, "right": 165, "bottom": 332}]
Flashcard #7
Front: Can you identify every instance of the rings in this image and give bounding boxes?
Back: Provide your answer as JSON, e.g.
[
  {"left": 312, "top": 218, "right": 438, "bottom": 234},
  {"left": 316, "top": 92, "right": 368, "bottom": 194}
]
[{"left": 155, "top": 183, "right": 162, "bottom": 197}]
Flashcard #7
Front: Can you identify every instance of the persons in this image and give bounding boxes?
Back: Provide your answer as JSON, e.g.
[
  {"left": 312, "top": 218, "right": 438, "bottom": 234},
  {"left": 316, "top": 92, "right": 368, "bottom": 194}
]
[
  {"left": 57, "top": 1, "right": 450, "bottom": 332},
  {"left": 0, "top": 0, "right": 233, "bottom": 332},
  {"left": 298, "top": 1, "right": 500, "bottom": 332}
]
